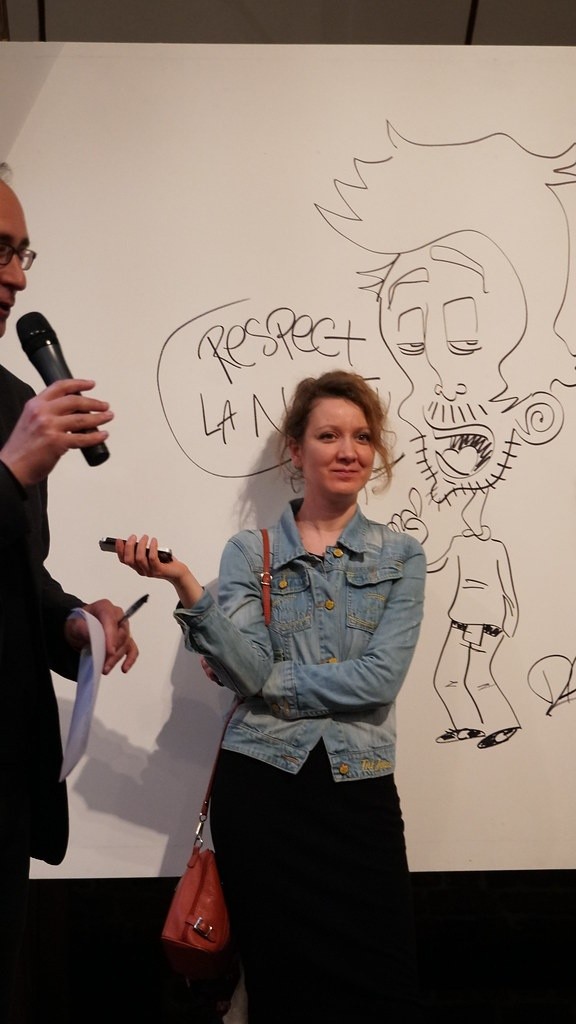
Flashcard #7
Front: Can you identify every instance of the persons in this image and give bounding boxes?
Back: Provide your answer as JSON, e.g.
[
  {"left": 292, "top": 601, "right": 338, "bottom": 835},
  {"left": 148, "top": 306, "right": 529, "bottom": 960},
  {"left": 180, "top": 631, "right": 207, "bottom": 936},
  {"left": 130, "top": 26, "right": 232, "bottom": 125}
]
[
  {"left": 116, "top": 369, "right": 425, "bottom": 1024},
  {"left": 0, "top": 162, "right": 140, "bottom": 1024}
]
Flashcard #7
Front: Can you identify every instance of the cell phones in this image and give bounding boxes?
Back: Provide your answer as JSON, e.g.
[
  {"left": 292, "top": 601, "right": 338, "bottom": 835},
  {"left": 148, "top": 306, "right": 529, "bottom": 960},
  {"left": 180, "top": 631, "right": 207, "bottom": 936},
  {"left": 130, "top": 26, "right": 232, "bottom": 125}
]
[{"left": 99, "top": 537, "right": 172, "bottom": 563}]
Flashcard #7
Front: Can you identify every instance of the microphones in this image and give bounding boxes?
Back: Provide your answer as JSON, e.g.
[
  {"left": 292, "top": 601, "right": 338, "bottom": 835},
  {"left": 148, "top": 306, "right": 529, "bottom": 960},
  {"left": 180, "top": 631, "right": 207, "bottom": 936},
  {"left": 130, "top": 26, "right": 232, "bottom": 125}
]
[{"left": 15, "top": 312, "right": 109, "bottom": 467}]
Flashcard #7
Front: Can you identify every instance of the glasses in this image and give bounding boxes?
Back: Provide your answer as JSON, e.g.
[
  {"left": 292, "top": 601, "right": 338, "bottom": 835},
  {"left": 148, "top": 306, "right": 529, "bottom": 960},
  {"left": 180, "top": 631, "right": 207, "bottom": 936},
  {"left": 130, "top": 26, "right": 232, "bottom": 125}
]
[{"left": 0, "top": 243, "right": 36, "bottom": 270}]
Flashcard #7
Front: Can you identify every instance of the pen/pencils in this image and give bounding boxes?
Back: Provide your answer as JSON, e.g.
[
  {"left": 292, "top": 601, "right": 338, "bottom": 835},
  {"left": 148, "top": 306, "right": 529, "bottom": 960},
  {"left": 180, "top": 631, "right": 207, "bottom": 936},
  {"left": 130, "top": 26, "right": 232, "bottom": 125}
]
[{"left": 116, "top": 593, "right": 151, "bottom": 628}]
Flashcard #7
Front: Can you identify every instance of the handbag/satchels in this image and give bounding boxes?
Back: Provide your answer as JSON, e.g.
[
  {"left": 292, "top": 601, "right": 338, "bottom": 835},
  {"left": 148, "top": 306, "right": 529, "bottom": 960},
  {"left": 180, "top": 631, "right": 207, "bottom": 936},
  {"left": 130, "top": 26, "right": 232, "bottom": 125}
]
[{"left": 160, "top": 849, "right": 235, "bottom": 977}]
